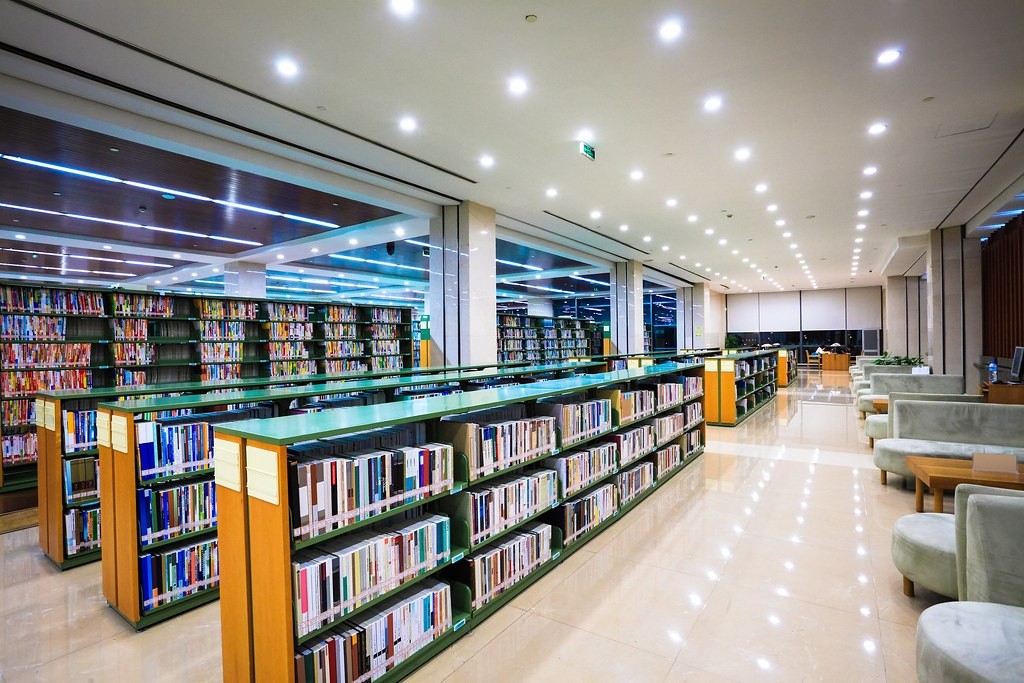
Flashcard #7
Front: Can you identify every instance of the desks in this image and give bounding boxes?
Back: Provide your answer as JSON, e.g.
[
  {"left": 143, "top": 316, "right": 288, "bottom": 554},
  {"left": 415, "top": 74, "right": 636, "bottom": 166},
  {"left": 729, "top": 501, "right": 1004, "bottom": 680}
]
[
  {"left": 905, "top": 456, "right": 1024, "bottom": 513},
  {"left": 872, "top": 399, "right": 888, "bottom": 414},
  {"left": 976, "top": 380, "right": 1024, "bottom": 404}
]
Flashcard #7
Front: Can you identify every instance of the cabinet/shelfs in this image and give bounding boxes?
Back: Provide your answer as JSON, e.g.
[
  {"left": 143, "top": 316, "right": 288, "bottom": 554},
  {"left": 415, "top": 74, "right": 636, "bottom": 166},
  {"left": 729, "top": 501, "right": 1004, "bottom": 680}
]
[{"left": 0, "top": 275, "right": 798, "bottom": 683}]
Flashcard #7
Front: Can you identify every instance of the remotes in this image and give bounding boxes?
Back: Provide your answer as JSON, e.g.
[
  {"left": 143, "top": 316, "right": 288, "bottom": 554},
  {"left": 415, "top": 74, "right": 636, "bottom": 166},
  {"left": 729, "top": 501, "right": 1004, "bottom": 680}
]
[{"left": 998, "top": 380, "right": 1007, "bottom": 384}]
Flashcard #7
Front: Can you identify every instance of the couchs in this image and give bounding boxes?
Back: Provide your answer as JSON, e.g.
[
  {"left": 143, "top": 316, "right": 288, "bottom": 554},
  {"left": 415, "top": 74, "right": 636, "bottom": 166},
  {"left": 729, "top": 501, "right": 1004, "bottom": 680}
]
[{"left": 849, "top": 357, "right": 1024, "bottom": 683}]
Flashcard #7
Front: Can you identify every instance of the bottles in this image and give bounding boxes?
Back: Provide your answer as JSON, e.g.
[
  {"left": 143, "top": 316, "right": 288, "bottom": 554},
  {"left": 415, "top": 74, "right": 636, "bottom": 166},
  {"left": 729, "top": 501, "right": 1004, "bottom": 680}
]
[{"left": 988, "top": 360, "right": 998, "bottom": 382}]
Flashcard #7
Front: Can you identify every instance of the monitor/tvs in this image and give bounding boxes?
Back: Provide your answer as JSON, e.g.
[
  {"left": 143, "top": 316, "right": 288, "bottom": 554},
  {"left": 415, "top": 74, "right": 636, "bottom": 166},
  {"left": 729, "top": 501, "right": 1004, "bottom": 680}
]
[{"left": 1007, "top": 346, "right": 1024, "bottom": 385}]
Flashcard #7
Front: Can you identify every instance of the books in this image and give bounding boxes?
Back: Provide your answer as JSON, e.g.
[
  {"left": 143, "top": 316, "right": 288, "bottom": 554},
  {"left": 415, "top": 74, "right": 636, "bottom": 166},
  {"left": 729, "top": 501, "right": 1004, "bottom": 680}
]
[
  {"left": 0, "top": 285, "right": 703, "bottom": 682},
  {"left": 735, "top": 349, "right": 797, "bottom": 416}
]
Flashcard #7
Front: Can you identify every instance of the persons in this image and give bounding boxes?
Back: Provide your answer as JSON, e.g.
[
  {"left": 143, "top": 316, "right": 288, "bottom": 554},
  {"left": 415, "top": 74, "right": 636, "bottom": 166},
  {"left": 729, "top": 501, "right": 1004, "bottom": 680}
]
[{"left": 815, "top": 345, "right": 829, "bottom": 367}]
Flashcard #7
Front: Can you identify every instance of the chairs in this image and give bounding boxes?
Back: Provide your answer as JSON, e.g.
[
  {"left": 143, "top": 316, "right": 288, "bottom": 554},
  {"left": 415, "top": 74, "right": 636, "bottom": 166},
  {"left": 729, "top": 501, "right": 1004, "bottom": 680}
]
[{"left": 806, "top": 350, "right": 820, "bottom": 370}]
